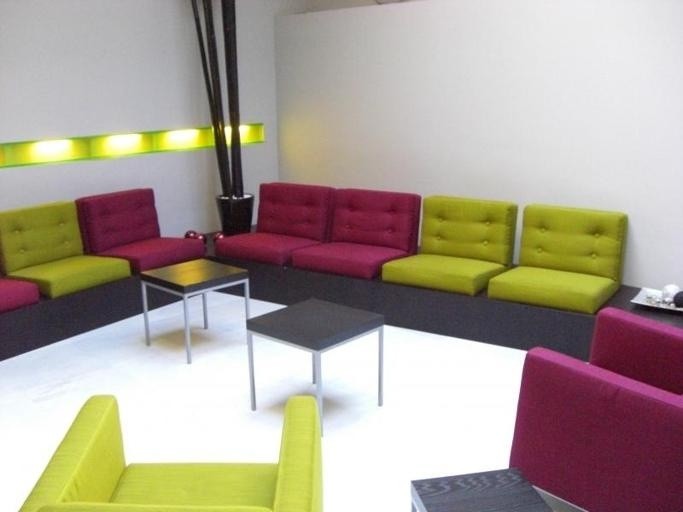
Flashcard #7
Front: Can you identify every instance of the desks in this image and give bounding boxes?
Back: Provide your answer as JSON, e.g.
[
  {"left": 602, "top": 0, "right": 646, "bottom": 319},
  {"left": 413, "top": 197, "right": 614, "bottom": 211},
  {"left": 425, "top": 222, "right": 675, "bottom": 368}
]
[
  {"left": 248, "top": 298, "right": 383, "bottom": 428},
  {"left": 137, "top": 259, "right": 249, "bottom": 363}
]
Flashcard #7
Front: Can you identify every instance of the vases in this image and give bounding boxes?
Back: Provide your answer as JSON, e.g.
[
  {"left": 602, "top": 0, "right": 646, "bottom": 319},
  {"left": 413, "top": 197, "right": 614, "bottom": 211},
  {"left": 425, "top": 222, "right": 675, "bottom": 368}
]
[{"left": 213, "top": 195, "right": 254, "bottom": 235}]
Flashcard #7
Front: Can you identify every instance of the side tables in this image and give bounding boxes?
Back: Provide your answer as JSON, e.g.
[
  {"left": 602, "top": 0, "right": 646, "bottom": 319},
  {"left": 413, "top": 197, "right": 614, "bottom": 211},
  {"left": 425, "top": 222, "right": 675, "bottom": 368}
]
[{"left": 407, "top": 468, "right": 553, "bottom": 511}]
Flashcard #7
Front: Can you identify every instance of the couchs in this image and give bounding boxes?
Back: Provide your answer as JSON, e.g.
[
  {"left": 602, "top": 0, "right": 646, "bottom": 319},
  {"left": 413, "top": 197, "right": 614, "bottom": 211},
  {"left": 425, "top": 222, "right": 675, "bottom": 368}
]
[
  {"left": 18, "top": 394, "right": 323, "bottom": 510},
  {"left": 485, "top": 202, "right": 628, "bottom": 374},
  {"left": 74, "top": 186, "right": 208, "bottom": 327},
  {"left": 215, "top": 182, "right": 330, "bottom": 309},
  {"left": 0, "top": 203, "right": 133, "bottom": 342},
  {"left": 509, "top": 308, "right": 682, "bottom": 511},
  {"left": 379, "top": 194, "right": 517, "bottom": 345},
  {"left": 289, "top": 188, "right": 420, "bottom": 337},
  {"left": 0, "top": 278, "right": 43, "bottom": 365}
]
